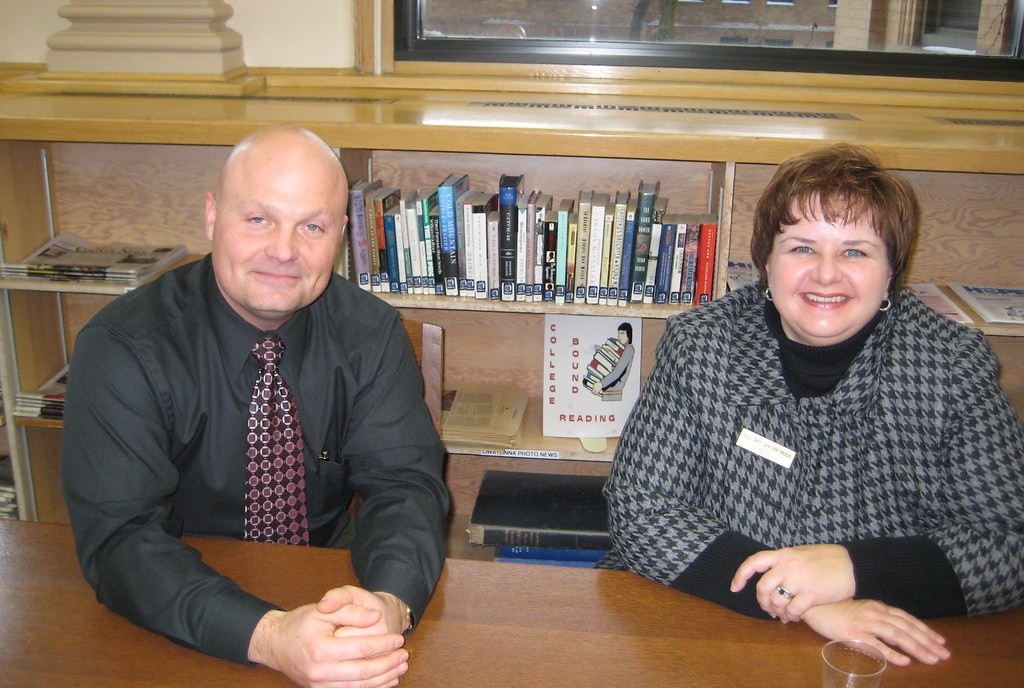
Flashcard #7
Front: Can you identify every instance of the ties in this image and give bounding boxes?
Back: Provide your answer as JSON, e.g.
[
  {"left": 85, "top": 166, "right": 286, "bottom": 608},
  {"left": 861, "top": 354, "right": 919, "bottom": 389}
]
[{"left": 244, "top": 333, "right": 313, "bottom": 546}]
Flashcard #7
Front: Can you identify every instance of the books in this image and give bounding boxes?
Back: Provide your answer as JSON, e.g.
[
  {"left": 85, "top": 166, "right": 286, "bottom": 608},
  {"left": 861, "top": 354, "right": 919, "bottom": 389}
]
[
  {"left": 347, "top": 174, "right": 718, "bottom": 307},
  {"left": 465, "top": 469, "right": 611, "bottom": 568}
]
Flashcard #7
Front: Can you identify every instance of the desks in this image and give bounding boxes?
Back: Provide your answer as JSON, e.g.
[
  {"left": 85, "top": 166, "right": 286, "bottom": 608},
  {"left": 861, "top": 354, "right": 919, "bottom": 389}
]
[{"left": 1, "top": 521, "right": 1024, "bottom": 688}]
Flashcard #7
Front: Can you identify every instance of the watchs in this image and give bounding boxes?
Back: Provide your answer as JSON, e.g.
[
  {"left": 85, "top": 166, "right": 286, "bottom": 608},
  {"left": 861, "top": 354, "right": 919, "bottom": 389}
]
[{"left": 405, "top": 604, "right": 415, "bottom": 630}]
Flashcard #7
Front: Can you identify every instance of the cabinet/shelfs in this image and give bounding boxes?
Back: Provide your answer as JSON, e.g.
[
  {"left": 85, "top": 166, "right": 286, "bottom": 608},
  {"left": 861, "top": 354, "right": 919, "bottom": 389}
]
[{"left": 0, "top": 63, "right": 1024, "bottom": 525}]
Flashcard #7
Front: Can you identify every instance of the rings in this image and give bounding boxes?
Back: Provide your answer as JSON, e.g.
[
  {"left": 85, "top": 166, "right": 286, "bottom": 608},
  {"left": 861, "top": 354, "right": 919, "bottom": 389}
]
[{"left": 777, "top": 584, "right": 793, "bottom": 600}]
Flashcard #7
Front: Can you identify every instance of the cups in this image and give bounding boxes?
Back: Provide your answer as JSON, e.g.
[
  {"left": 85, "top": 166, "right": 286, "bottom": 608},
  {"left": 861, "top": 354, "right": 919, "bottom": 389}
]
[{"left": 820, "top": 638, "right": 888, "bottom": 688}]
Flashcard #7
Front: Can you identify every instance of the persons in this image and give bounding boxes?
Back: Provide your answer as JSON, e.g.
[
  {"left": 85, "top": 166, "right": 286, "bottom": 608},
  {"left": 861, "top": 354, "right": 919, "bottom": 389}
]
[
  {"left": 62, "top": 124, "right": 449, "bottom": 688},
  {"left": 602, "top": 140, "right": 1023, "bottom": 665}
]
